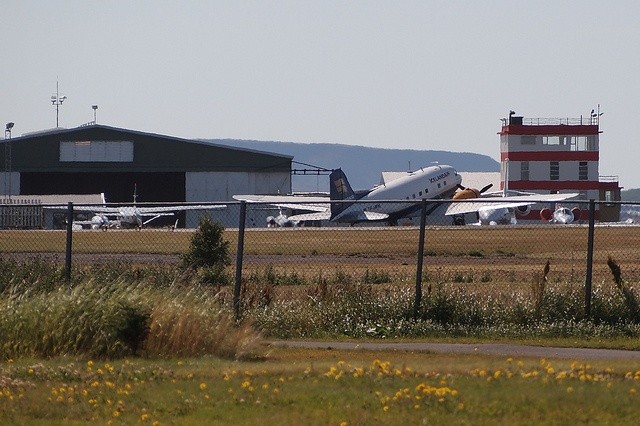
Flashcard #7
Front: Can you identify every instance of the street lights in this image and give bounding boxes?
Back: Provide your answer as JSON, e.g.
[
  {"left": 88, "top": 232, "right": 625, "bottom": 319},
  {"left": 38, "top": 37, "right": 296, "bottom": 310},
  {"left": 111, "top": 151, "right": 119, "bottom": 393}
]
[{"left": 4, "top": 118, "right": 14, "bottom": 198}]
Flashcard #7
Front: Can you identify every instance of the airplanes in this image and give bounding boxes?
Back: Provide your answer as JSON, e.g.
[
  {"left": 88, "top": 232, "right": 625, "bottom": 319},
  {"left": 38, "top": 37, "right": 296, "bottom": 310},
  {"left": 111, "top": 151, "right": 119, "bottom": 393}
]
[
  {"left": 39, "top": 200, "right": 228, "bottom": 231},
  {"left": 233, "top": 161, "right": 579, "bottom": 226},
  {"left": 540, "top": 206, "right": 581, "bottom": 225}
]
